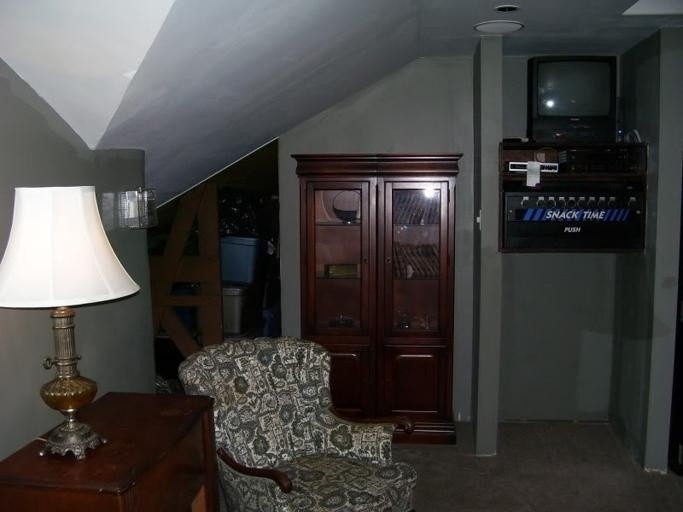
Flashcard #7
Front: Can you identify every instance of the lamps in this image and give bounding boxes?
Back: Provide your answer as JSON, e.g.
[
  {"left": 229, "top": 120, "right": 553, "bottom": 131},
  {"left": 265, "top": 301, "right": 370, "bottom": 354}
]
[{"left": 1, "top": 185, "right": 143, "bottom": 462}]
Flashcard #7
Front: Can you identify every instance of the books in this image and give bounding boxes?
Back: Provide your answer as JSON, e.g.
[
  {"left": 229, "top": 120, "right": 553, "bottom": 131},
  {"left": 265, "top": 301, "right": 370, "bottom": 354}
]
[{"left": 392, "top": 190, "right": 440, "bottom": 279}]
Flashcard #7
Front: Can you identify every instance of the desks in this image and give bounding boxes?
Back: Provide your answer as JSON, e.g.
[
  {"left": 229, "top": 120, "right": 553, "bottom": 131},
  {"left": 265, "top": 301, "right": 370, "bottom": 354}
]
[{"left": 0, "top": 391, "right": 221, "bottom": 512}]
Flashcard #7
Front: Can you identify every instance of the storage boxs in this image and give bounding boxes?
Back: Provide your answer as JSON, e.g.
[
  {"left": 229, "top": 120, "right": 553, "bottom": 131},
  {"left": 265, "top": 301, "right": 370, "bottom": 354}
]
[{"left": 222, "top": 236, "right": 261, "bottom": 334}]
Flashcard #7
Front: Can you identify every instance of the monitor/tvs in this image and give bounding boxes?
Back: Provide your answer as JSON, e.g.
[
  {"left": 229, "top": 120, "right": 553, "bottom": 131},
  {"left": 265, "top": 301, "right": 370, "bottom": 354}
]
[{"left": 525, "top": 53, "right": 618, "bottom": 143}]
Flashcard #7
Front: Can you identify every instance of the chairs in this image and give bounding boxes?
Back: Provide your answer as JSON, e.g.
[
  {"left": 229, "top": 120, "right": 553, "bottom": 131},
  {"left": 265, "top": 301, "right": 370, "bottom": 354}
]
[{"left": 176, "top": 334, "right": 419, "bottom": 512}]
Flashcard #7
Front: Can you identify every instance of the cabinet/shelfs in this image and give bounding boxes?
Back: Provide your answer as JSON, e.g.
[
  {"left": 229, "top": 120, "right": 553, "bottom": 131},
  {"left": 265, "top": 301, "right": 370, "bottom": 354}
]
[
  {"left": 497, "top": 138, "right": 647, "bottom": 254},
  {"left": 290, "top": 151, "right": 466, "bottom": 447}
]
